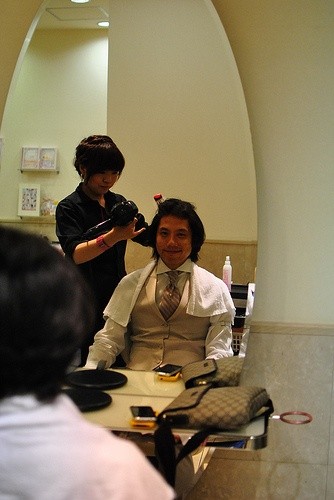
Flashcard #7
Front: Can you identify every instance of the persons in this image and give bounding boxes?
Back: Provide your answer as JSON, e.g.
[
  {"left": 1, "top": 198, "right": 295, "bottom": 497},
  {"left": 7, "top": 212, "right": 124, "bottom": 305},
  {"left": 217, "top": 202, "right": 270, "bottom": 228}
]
[
  {"left": 56, "top": 135, "right": 160, "bottom": 368},
  {"left": 0, "top": 228, "right": 179, "bottom": 500},
  {"left": 82, "top": 198, "right": 235, "bottom": 373}
]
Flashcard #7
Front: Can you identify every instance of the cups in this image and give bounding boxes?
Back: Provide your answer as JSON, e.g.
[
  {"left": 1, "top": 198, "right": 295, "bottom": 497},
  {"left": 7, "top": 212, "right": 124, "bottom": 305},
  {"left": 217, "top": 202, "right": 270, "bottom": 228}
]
[{"left": 49, "top": 205, "right": 57, "bottom": 215}]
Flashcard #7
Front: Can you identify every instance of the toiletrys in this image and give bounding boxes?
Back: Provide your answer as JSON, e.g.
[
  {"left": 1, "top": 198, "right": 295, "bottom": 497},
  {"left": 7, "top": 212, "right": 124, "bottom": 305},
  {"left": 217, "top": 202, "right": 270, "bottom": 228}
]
[{"left": 222, "top": 255, "right": 232, "bottom": 292}]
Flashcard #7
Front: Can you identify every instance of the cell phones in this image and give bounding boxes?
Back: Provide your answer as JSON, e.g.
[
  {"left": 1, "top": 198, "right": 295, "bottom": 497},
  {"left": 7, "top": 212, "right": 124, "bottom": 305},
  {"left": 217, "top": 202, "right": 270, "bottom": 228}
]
[
  {"left": 154, "top": 364, "right": 182, "bottom": 376},
  {"left": 131, "top": 405, "right": 157, "bottom": 420}
]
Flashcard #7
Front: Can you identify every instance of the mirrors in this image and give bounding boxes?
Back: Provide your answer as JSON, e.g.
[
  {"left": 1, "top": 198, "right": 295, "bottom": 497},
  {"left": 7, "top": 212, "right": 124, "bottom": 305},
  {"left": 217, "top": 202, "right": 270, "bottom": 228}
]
[{"left": 0, "top": 0, "right": 258, "bottom": 397}]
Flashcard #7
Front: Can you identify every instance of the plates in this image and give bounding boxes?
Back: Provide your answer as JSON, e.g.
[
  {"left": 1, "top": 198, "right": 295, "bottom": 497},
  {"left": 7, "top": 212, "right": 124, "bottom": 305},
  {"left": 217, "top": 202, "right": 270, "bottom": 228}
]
[
  {"left": 65, "top": 370, "right": 128, "bottom": 391},
  {"left": 62, "top": 388, "right": 113, "bottom": 412}
]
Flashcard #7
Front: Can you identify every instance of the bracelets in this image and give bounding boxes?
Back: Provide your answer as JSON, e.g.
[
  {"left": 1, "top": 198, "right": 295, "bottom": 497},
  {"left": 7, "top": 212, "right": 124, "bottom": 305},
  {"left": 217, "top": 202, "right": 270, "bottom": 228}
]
[{"left": 96, "top": 235, "right": 110, "bottom": 251}]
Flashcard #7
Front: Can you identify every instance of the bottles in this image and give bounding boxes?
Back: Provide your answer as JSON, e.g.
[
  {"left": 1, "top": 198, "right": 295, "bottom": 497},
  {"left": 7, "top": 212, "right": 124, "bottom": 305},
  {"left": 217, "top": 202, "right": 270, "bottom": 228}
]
[{"left": 223, "top": 255, "right": 232, "bottom": 292}]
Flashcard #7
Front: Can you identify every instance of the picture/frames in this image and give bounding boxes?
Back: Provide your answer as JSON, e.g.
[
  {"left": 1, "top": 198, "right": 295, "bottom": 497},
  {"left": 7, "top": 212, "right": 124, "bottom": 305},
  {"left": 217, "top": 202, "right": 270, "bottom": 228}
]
[
  {"left": 17, "top": 183, "right": 41, "bottom": 216},
  {"left": 21, "top": 147, "right": 57, "bottom": 169}
]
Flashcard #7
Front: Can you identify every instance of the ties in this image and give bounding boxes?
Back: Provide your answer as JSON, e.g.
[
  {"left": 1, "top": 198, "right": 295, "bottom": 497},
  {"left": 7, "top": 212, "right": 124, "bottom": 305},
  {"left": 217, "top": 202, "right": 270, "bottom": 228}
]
[
  {"left": 159, "top": 270, "right": 181, "bottom": 320},
  {"left": 180, "top": 357, "right": 245, "bottom": 386},
  {"left": 156, "top": 384, "right": 268, "bottom": 431}
]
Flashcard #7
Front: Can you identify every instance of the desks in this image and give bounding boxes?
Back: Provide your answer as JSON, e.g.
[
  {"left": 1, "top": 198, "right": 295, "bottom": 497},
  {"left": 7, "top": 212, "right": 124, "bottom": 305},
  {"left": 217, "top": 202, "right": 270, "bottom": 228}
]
[
  {"left": 81, "top": 393, "right": 265, "bottom": 437},
  {"left": 61, "top": 368, "right": 186, "bottom": 397}
]
